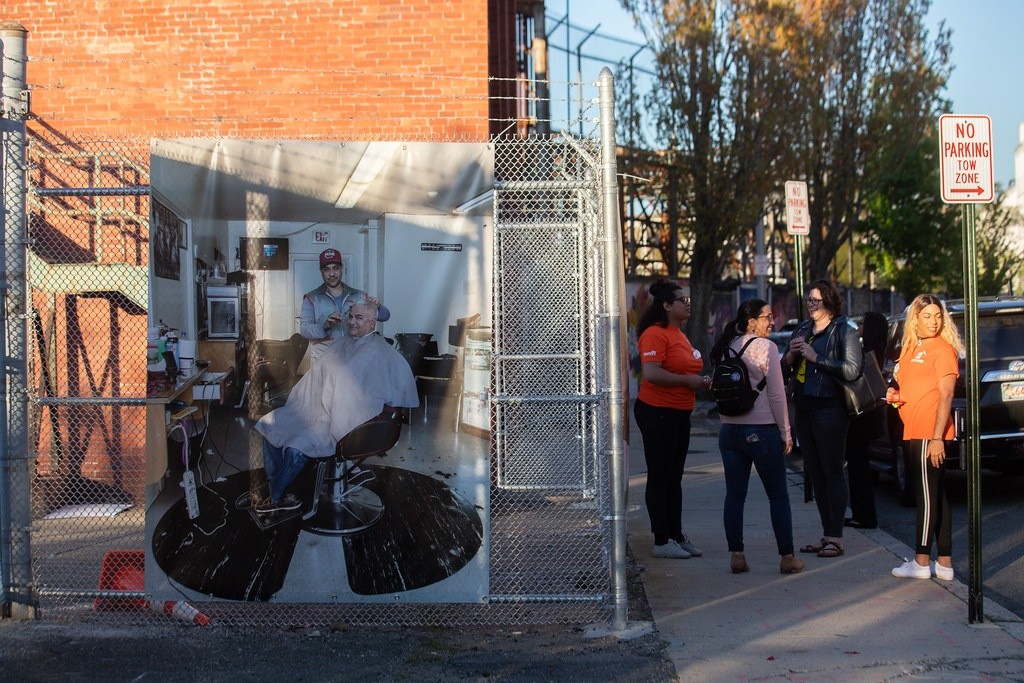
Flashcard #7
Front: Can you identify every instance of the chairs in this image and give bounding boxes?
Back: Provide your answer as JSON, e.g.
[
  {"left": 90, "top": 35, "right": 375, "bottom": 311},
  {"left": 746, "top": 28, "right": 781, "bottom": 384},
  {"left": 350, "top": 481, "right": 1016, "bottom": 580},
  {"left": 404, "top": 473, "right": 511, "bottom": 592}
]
[{"left": 244, "top": 333, "right": 459, "bottom": 534}]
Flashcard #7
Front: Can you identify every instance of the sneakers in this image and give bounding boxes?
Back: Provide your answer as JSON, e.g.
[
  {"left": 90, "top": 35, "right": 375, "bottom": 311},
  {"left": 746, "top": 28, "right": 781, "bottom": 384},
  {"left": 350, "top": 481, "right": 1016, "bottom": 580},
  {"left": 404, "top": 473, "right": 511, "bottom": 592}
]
[
  {"left": 677, "top": 539, "right": 704, "bottom": 558},
  {"left": 654, "top": 539, "right": 691, "bottom": 558},
  {"left": 930, "top": 561, "right": 954, "bottom": 580},
  {"left": 891, "top": 557, "right": 931, "bottom": 579}
]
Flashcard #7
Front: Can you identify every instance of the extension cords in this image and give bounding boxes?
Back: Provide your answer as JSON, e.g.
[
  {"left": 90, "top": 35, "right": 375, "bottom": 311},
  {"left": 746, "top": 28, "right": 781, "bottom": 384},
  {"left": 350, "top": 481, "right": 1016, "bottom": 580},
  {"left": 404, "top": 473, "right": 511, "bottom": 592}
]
[{"left": 183, "top": 471, "right": 199, "bottom": 520}]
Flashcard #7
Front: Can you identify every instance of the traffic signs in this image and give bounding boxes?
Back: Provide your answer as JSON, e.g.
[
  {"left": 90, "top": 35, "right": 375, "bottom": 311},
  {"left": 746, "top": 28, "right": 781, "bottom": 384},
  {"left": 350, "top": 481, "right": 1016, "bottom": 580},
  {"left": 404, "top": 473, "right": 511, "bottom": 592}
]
[
  {"left": 939, "top": 113, "right": 995, "bottom": 203},
  {"left": 785, "top": 180, "right": 810, "bottom": 236}
]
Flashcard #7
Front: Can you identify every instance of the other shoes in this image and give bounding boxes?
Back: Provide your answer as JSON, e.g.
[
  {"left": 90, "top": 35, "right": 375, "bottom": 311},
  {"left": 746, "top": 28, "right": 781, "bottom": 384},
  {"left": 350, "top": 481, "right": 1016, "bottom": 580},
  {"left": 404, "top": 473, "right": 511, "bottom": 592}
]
[
  {"left": 732, "top": 553, "right": 749, "bottom": 573},
  {"left": 781, "top": 557, "right": 805, "bottom": 573}
]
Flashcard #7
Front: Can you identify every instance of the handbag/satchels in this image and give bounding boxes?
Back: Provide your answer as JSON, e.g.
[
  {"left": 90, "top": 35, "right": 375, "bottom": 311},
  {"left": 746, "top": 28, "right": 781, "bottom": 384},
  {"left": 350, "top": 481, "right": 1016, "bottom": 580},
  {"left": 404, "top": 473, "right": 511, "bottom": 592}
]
[{"left": 833, "top": 351, "right": 889, "bottom": 415}]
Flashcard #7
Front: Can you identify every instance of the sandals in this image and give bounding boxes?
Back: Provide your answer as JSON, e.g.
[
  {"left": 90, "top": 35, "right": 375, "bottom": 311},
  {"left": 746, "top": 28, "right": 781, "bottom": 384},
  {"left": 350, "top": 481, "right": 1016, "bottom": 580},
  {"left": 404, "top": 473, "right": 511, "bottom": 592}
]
[
  {"left": 801, "top": 541, "right": 822, "bottom": 553},
  {"left": 843, "top": 517, "right": 877, "bottom": 529},
  {"left": 817, "top": 542, "right": 844, "bottom": 557}
]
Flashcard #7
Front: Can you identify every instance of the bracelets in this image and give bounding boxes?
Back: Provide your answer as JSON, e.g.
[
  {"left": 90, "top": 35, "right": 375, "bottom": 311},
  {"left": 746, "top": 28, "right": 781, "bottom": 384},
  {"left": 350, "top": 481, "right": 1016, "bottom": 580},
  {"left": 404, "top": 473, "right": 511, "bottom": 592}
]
[{"left": 932, "top": 437, "right": 943, "bottom": 441}]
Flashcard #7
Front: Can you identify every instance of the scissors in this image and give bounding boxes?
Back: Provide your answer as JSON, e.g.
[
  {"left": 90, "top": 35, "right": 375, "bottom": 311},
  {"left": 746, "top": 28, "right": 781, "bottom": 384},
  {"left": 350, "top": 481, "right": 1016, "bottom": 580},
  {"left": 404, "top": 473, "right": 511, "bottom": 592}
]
[{"left": 336, "top": 307, "right": 351, "bottom": 322}]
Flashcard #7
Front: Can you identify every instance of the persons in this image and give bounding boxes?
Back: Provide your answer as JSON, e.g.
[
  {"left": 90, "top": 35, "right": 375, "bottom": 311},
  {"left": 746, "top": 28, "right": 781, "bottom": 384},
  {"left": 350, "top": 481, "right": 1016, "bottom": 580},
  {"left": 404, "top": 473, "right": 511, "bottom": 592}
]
[
  {"left": 886, "top": 296, "right": 964, "bottom": 580},
  {"left": 845, "top": 310, "right": 888, "bottom": 529},
  {"left": 781, "top": 281, "right": 865, "bottom": 556},
  {"left": 634, "top": 281, "right": 713, "bottom": 560},
  {"left": 711, "top": 297, "right": 805, "bottom": 574},
  {"left": 254, "top": 297, "right": 421, "bottom": 512},
  {"left": 301, "top": 248, "right": 390, "bottom": 474}
]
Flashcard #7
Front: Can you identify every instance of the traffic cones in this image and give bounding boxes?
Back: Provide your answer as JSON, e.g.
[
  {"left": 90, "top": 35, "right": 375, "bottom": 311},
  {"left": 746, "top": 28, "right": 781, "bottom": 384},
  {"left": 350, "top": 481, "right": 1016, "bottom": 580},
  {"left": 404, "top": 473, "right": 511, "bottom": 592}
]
[{"left": 95, "top": 548, "right": 208, "bottom": 627}]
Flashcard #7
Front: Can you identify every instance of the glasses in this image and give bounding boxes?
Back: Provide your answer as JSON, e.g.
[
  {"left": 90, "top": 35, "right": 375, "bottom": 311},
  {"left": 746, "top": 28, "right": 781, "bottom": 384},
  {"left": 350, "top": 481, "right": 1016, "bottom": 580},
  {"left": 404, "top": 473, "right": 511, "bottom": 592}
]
[
  {"left": 672, "top": 297, "right": 691, "bottom": 304},
  {"left": 857, "top": 320, "right": 864, "bottom": 326},
  {"left": 758, "top": 314, "right": 773, "bottom": 321},
  {"left": 805, "top": 298, "right": 823, "bottom": 305}
]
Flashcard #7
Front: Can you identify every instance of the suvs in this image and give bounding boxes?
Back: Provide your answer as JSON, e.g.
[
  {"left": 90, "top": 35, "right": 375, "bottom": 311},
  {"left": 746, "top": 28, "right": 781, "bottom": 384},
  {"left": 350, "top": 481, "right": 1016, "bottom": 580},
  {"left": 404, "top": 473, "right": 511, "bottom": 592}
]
[{"left": 853, "top": 294, "right": 1024, "bottom": 510}]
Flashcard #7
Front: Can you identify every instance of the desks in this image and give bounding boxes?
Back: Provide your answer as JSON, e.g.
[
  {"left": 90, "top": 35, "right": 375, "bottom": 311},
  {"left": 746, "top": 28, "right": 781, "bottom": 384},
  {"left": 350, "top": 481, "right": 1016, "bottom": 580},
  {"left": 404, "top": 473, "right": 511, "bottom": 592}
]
[{"left": 146, "top": 361, "right": 232, "bottom": 508}]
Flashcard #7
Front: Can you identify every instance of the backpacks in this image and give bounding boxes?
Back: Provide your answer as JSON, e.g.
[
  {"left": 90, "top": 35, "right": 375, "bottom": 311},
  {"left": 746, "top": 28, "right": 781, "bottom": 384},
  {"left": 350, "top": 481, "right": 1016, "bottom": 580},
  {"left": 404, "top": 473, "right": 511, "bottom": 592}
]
[{"left": 711, "top": 337, "right": 767, "bottom": 416}]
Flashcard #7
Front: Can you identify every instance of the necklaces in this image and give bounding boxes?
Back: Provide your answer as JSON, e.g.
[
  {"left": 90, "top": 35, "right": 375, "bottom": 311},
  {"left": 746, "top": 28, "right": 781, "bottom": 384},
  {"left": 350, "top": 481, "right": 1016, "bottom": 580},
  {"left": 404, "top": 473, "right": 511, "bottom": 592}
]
[{"left": 813, "top": 326, "right": 820, "bottom": 333}]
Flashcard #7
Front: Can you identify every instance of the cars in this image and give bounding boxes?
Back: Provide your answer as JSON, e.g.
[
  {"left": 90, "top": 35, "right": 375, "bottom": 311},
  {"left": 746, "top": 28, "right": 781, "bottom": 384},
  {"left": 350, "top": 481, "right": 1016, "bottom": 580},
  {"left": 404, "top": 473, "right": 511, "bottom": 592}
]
[{"left": 768, "top": 313, "right": 888, "bottom": 370}]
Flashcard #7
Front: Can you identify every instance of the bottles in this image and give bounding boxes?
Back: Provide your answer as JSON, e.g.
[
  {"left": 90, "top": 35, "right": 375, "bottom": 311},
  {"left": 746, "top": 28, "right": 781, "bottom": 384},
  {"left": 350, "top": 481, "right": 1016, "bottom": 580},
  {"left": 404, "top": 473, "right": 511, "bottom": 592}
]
[{"left": 148, "top": 319, "right": 189, "bottom": 371}]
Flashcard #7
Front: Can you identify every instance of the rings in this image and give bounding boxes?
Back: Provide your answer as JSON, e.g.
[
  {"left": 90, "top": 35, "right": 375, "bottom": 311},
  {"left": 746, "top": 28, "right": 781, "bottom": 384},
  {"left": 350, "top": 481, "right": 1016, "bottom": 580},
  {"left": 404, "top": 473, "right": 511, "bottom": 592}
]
[{"left": 789, "top": 448, "right": 792, "bottom": 449}]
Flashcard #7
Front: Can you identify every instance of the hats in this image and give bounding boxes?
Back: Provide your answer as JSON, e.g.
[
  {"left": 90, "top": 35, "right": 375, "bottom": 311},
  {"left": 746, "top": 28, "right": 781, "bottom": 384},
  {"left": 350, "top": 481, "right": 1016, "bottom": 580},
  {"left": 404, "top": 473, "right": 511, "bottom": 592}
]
[{"left": 321, "top": 248, "right": 342, "bottom": 266}]
